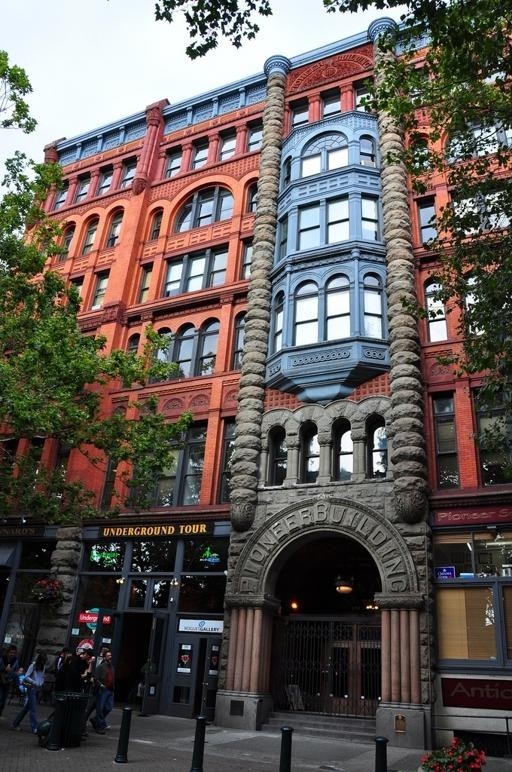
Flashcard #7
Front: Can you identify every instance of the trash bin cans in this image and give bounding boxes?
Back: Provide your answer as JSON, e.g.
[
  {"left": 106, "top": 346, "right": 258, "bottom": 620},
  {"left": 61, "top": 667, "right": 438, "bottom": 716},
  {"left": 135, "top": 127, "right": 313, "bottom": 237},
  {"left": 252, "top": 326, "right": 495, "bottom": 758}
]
[{"left": 50, "top": 691, "right": 94, "bottom": 747}]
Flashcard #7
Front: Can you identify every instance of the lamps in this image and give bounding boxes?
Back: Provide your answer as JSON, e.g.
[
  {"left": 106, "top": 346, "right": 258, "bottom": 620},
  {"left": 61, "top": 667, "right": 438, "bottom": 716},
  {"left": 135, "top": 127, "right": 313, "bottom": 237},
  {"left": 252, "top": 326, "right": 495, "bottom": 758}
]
[{"left": 336, "top": 575, "right": 356, "bottom": 594}]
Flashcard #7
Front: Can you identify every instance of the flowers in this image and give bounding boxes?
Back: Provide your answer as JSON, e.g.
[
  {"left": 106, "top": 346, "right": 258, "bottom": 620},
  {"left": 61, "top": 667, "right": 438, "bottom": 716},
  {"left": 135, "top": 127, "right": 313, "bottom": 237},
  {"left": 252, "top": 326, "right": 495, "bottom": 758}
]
[
  {"left": 32, "top": 579, "right": 65, "bottom": 604},
  {"left": 419, "top": 735, "right": 487, "bottom": 771}
]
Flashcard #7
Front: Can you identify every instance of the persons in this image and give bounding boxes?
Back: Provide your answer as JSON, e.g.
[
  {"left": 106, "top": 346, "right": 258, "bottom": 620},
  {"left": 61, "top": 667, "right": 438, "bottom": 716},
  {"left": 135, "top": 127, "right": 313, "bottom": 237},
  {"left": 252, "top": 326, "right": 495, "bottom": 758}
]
[
  {"left": 1, "top": 644, "right": 115, "bottom": 737},
  {"left": 144, "top": 654, "right": 157, "bottom": 684}
]
